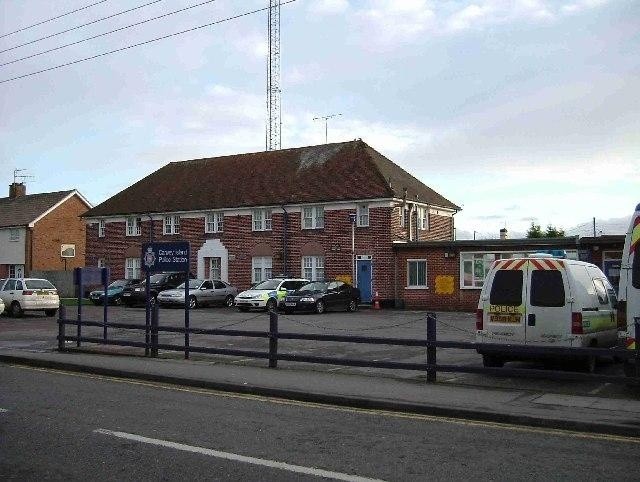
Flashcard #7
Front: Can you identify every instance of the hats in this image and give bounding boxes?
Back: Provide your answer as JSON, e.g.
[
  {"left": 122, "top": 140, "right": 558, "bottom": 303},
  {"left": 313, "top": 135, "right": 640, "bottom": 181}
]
[
  {"left": 158, "top": 278, "right": 237, "bottom": 310},
  {"left": 282, "top": 281, "right": 362, "bottom": 314},
  {"left": 0, "top": 277, "right": 60, "bottom": 317},
  {"left": 234, "top": 278, "right": 310, "bottom": 312},
  {"left": 88, "top": 278, "right": 143, "bottom": 305}
]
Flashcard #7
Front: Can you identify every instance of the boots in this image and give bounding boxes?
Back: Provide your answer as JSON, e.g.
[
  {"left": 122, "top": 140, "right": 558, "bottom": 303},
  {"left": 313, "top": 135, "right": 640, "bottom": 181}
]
[{"left": 122, "top": 272, "right": 194, "bottom": 308}]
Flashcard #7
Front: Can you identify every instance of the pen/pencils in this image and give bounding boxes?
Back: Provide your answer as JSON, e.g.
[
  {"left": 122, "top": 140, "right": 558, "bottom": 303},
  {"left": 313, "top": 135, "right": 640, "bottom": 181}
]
[
  {"left": 616, "top": 200, "right": 638, "bottom": 383},
  {"left": 474, "top": 254, "right": 619, "bottom": 371}
]
